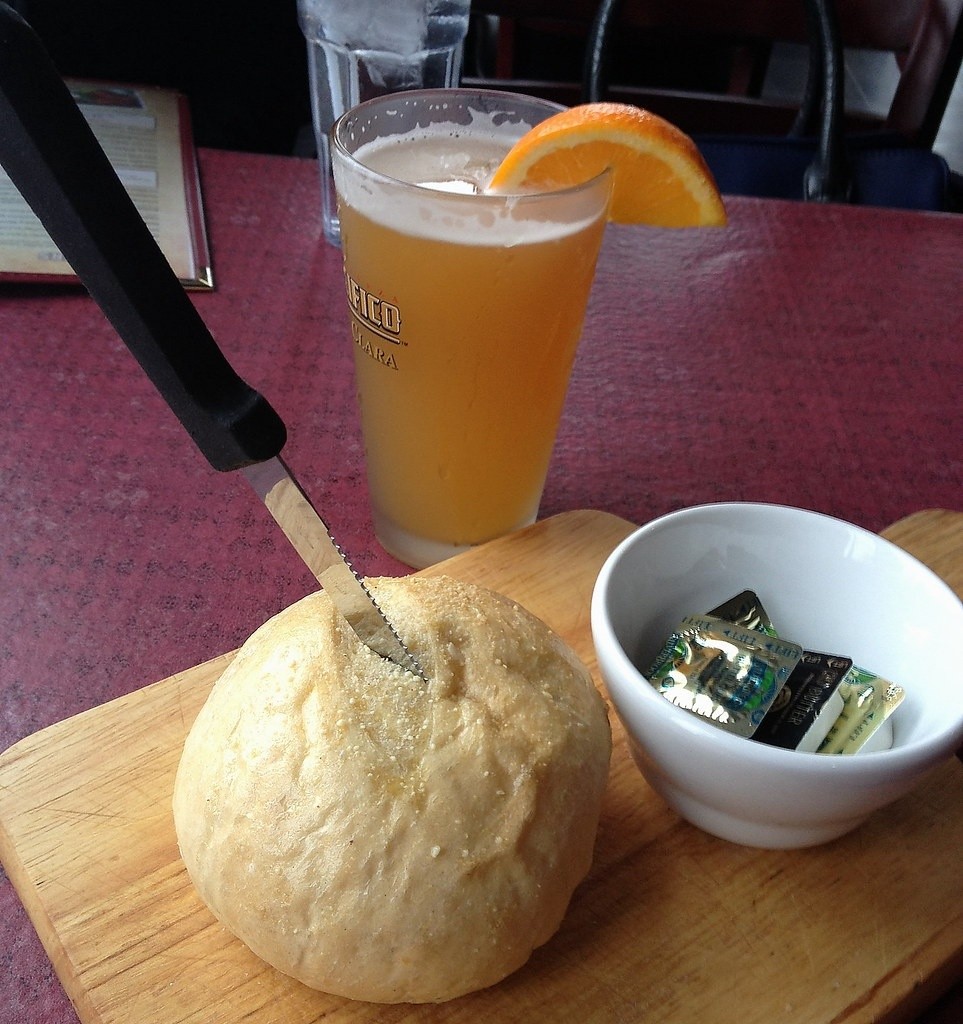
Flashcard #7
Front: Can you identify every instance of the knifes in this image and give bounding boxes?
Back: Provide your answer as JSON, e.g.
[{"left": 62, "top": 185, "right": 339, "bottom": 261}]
[{"left": 0, "top": 0, "right": 427, "bottom": 684}]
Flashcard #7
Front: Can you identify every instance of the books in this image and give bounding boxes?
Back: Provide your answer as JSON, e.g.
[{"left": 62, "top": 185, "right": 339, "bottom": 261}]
[{"left": 0, "top": 69, "right": 216, "bottom": 293}]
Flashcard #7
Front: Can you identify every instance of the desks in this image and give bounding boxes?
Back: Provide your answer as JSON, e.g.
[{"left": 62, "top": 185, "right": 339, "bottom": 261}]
[{"left": 0, "top": 147, "right": 963, "bottom": 1024}]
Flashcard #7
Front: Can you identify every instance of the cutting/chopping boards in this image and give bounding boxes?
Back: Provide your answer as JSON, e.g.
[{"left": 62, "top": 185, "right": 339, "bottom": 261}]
[{"left": 0, "top": 508, "right": 963, "bottom": 1024}]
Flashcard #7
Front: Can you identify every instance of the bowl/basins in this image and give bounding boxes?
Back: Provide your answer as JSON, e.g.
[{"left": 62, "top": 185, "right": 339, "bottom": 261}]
[{"left": 590, "top": 502, "right": 963, "bottom": 850}]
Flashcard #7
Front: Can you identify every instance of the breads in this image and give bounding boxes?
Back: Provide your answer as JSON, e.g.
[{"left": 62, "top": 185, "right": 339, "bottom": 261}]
[{"left": 174, "top": 574, "right": 612, "bottom": 1003}]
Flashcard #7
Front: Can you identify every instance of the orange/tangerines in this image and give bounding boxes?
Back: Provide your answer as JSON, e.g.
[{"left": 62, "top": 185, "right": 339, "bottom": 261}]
[{"left": 489, "top": 102, "right": 728, "bottom": 228}]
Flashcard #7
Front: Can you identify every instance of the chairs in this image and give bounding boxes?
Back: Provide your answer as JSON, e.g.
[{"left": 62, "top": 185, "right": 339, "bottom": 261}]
[{"left": 587, "top": 0, "right": 849, "bottom": 205}]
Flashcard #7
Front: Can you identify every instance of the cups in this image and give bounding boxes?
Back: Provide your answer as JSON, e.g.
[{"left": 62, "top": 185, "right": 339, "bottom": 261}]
[
  {"left": 298, "top": 0, "right": 471, "bottom": 249},
  {"left": 326, "top": 88, "right": 614, "bottom": 571}
]
